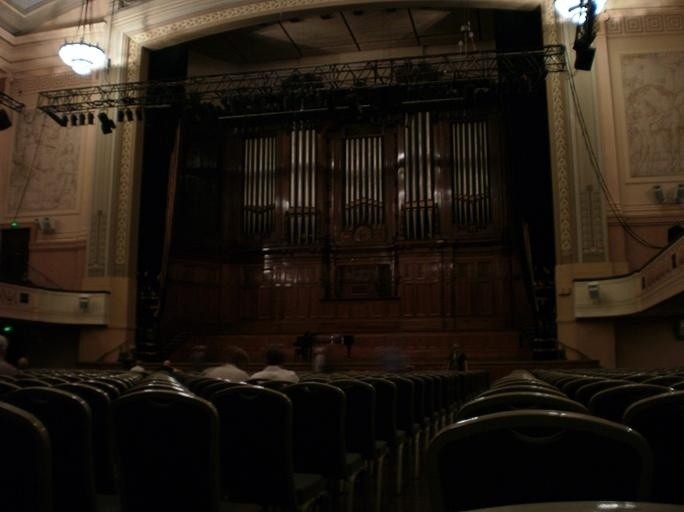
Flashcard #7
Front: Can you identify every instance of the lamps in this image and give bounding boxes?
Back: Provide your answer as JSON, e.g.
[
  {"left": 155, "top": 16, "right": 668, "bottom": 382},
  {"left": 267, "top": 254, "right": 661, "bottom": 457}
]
[
  {"left": 60, "top": 106, "right": 143, "bottom": 134},
  {"left": 0, "top": 93, "right": 26, "bottom": 130},
  {"left": 565, "top": 1, "right": 602, "bottom": 72},
  {"left": 550, "top": 1, "right": 609, "bottom": 25},
  {"left": 56, "top": 0, "right": 110, "bottom": 76}
]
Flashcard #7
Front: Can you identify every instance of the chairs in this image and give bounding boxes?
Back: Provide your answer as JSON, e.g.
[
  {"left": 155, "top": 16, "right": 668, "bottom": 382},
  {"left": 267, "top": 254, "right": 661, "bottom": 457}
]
[{"left": 0, "top": 364, "right": 683, "bottom": 512}]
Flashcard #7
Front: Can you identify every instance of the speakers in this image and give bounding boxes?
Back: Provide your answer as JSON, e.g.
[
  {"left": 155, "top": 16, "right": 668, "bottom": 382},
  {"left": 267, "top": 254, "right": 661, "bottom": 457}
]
[
  {"left": 0, "top": 108, "right": 11, "bottom": 130},
  {"left": 575, "top": 46, "right": 596, "bottom": 72}
]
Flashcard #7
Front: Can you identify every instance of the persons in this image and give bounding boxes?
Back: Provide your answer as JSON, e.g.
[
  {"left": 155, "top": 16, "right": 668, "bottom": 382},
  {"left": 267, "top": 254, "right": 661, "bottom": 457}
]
[
  {"left": 248, "top": 344, "right": 300, "bottom": 384},
  {"left": 0, "top": 335, "right": 23, "bottom": 377},
  {"left": 447, "top": 343, "right": 468, "bottom": 372},
  {"left": 157, "top": 360, "right": 184, "bottom": 374},
  {"left": 201, "top": 347, "right": 249, "bottom": 381},
  {"left": 130, "top": 360, "right": 145, "bottom": 372},
  {"left": 310, "top": 343, "right": 357, "bottom": 376},
  {"left": 18, "top": 358, "right": 29, "bottom": 369}
]
